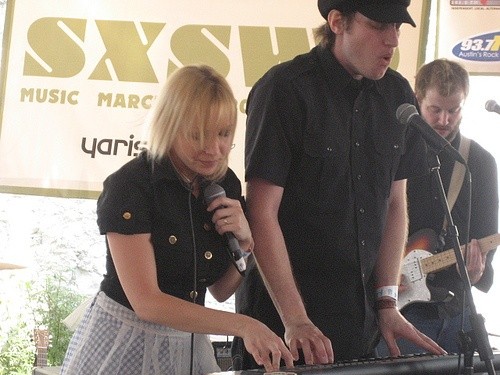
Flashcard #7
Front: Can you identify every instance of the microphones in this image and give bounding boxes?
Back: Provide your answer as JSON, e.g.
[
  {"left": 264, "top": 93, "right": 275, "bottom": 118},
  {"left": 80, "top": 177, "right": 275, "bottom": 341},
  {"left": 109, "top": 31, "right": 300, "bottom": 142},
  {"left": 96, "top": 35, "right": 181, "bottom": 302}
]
[
  {"left": 485, "top": 100, "right": 500, "bottom": 114},
  {"left": 396, "top": 103, "right": 465, "bottom": 164},
  {"left": 204, "top": 184, "right": 247, "bottom": 277}
]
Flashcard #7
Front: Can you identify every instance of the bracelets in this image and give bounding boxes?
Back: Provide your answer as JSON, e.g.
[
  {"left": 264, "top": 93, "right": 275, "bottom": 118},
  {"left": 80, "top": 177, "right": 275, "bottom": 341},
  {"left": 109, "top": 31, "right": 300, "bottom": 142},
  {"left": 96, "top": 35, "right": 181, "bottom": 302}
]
[
  {"left": 240, "top": 239, "right": 254, "bottom": 258},
  {"left": 375, "top": 285, "right": 399, "bottom": 300},
  {"left": 376, "top": 299, "right": 396, "bottom": 310}
]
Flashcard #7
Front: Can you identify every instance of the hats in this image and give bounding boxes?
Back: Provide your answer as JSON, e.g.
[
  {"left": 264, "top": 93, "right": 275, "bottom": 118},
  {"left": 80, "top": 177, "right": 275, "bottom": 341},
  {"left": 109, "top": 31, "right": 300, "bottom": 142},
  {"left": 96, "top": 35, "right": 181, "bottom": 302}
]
[{"left": 317, "top": 0, "right": 417, "bottom": 28}]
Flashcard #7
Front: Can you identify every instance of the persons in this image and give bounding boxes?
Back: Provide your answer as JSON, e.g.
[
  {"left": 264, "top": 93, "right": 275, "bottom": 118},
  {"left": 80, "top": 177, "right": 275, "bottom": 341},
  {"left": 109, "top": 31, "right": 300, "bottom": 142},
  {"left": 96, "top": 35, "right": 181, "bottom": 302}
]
[
  {"left": 230, "top": 0, "right": 449, "bottom": 371},
  {"left": 60, "top": 65, "right": 294, "bottom": 375},
  {"left": 377, "top": 58, "right": 499, "bottom": 357}
]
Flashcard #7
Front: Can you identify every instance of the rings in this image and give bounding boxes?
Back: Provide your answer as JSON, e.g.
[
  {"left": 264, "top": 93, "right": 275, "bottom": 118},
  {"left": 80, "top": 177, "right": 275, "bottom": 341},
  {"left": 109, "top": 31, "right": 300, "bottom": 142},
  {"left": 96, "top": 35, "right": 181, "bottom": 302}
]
[{"left": 224, "top": 219, "right": 230, "bottom": 224}]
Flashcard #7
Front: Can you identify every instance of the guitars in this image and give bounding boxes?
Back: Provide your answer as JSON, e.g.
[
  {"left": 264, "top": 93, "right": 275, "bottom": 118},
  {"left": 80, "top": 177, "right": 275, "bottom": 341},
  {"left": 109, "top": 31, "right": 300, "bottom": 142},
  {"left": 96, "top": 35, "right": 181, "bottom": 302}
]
[
  {"left": 29, "top": 328, "right": 49, "bottom": 367},
  {"left": 397, "top": 225, "right": 500, "bottom": 313}
]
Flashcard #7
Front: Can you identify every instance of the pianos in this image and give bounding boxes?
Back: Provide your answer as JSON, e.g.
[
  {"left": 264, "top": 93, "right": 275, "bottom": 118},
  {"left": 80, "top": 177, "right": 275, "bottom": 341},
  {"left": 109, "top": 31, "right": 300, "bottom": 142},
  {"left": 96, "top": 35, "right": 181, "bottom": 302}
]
[{"left": 205, "top": 347, "right": 500, "bottom": 375}]
